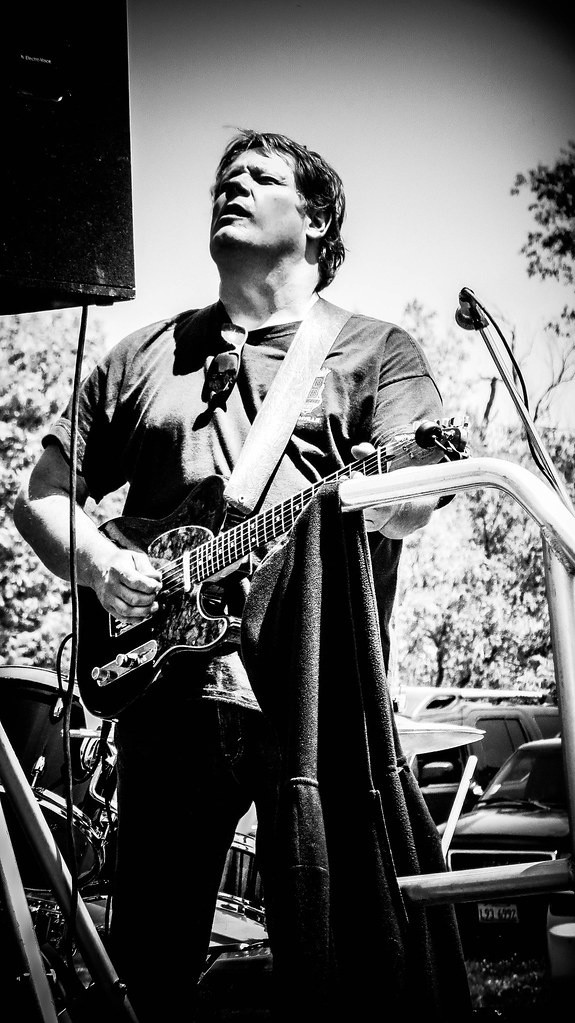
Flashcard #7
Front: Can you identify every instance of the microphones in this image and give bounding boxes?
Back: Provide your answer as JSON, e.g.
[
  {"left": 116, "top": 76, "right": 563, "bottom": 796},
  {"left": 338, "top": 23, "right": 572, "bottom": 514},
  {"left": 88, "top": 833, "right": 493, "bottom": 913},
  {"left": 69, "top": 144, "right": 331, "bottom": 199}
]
[{"left": 455, "top": 287, "right": 484, "bottom": 330}]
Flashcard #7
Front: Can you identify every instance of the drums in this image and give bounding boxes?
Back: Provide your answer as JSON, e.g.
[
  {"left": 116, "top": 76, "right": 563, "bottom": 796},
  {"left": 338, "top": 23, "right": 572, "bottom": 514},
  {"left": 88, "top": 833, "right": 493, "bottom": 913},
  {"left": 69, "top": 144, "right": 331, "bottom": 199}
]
[
  {"left": 0, "top": 664, "right": 121, "bottom": 894},
  {"left": 209, "top": 831, "right": 270, "bottom": 945},
  {"left": 0, "top": 896, "right": 67, "bottom": 975}
]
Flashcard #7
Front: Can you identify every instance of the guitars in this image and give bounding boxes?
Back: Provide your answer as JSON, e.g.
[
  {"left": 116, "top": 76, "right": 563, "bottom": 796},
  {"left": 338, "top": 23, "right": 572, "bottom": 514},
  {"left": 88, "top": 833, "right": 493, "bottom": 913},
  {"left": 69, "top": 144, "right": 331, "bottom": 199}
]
[{"left": 73, "top": 412, "right": 471, "bottom": 722}]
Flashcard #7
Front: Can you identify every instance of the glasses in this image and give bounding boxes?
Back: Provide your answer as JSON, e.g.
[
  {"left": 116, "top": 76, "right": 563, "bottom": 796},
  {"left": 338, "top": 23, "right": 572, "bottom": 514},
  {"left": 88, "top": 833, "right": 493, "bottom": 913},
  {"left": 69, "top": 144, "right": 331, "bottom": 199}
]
[{"left": 204, "top": 323, "right": 248, "bottom": 393}]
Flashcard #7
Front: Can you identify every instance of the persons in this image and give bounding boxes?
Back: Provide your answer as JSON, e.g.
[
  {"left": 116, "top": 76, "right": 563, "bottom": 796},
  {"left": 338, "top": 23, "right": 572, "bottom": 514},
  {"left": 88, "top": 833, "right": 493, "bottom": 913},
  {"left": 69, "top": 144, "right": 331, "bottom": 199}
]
[{"left": 13, "top": 128, "right": 446, "bottom": 1023}]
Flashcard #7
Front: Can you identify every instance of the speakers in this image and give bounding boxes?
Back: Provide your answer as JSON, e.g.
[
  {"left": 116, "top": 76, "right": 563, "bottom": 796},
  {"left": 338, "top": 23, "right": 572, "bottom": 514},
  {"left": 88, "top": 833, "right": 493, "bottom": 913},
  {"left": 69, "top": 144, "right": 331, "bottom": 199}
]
[{"left": 0, "top": 0, "right": 138, "bottom": 315}]
